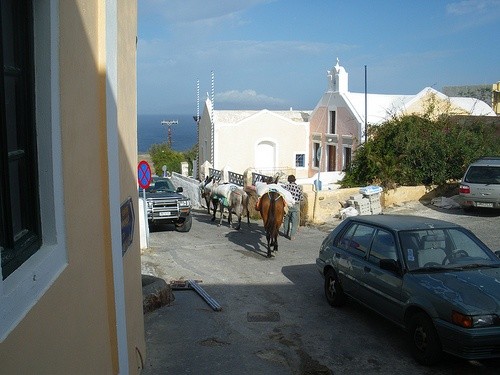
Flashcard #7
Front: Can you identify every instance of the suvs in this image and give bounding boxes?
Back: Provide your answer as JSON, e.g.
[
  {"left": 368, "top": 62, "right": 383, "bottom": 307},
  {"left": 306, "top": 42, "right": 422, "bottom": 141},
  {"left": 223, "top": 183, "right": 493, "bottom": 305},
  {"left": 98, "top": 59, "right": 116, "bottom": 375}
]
[{"left": 138, "top": 176, "right": 193, "bottom": 232}]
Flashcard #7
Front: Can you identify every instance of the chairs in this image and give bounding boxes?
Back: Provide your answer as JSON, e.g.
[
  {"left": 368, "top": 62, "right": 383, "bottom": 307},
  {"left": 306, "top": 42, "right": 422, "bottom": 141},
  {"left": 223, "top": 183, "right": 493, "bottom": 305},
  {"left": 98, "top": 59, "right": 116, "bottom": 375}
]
[{"left": 365, "top": 232, "right": 450, "bottom": 272}]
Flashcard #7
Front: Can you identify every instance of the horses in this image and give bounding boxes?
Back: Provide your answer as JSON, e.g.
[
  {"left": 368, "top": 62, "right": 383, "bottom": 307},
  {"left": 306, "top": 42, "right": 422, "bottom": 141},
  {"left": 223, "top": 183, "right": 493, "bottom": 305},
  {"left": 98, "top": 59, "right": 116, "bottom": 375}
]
[
  {"left": 199, "top": 175, "right": 233, "bottom": 224},
  {"left": 259, "top": 175, "right": 285, "bottom": 258},
  {"left": 208, "top": 176, "right": 250, "bottom": 230}
]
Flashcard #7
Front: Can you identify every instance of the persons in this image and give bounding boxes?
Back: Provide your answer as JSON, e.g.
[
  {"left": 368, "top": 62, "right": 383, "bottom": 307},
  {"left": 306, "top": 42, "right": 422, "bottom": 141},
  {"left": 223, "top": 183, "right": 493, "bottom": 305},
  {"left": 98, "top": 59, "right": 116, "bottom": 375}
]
[{"left": 281, "top": 175, "right": 305, "bottom": 240}]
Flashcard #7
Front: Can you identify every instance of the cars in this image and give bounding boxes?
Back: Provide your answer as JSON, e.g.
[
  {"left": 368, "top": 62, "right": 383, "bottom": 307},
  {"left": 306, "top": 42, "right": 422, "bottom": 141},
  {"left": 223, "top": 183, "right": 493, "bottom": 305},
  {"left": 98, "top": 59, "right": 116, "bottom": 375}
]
[
  {"left": 458, "top": 157, "right": 500, "bottom": 212},
  {"left": 314, "top": 211, "right": 500, "bottom": 363}
]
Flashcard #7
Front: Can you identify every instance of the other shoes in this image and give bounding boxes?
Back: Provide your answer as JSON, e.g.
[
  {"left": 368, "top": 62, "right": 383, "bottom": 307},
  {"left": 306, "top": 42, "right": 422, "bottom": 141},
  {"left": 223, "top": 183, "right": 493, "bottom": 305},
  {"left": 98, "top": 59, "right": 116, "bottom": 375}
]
[
  {"left": 290, "top": 236, "right": 296, "bottom": 240},
  {"left": 284, "top": 234, "right": 288, "bottom": 238}
]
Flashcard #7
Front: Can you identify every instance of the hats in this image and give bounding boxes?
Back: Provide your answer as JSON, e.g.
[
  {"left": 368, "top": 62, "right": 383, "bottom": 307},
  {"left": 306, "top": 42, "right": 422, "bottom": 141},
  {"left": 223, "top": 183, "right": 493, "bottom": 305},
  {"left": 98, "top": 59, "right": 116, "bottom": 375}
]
[{"left": 288, "top": 175, "right": 296, "bottom": 182}]
